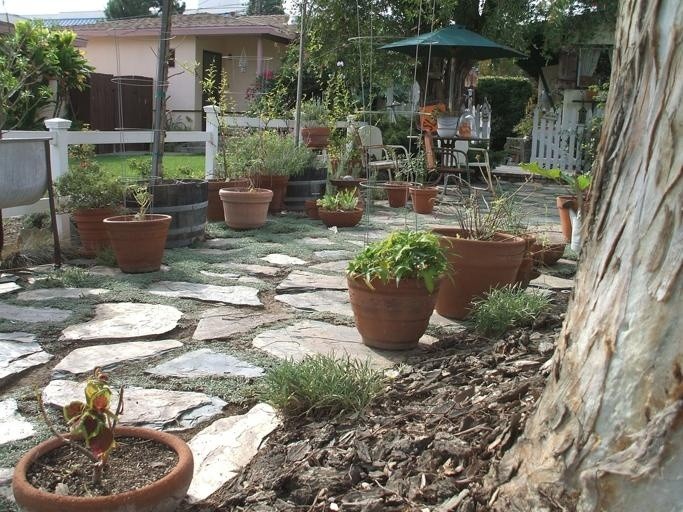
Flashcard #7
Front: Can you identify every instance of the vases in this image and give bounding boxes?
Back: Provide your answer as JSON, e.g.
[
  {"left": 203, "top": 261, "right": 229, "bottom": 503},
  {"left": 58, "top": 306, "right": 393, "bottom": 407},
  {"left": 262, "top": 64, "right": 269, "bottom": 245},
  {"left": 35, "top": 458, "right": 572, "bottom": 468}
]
[
  {"left": 507, "top": 237, "right": 538, "bottom": 290},
  {"left": 409, "top": 153, "right": 438, "bottom": 214},
  {"left": 534, "top": 243, "right": 566, "bottom": 265}
]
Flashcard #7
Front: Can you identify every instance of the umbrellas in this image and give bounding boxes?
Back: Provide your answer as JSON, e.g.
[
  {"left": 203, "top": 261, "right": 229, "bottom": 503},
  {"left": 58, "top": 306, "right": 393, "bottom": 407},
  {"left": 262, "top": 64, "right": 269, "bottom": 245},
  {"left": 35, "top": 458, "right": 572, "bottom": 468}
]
[{"left": 374, "top": 24, "right": 531, "bottom": 111}]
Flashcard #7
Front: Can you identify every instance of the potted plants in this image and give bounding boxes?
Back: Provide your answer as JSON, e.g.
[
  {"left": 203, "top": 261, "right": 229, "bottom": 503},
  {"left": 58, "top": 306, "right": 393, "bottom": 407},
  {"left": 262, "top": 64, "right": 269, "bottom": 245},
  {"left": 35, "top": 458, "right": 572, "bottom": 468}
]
[
  {"left": 173, "top": 62, "right": 368, "bottom": 230},
  {"left": 431, "top": 107, "right": 459, "bottom": 138},
  {"left": 345, "top": 230, "right": 463, "bottom": 351},
  {"left": 102, "top": 157, "right": 170, "bottom": 274},
  {"left": 53, "top": 144, "right": 129, "bottom": 260},
  {"left": 556, "top": 170, "right": 591, "bottom": 244},
  {"left": 13, "top": 366, "right": 193, "bottom": 512},
  {"left": 383, "top": 144, "right": 413, "bottom": 208},
  {"left": 0, "top": 18, "right": 96, "bottom": 208},
  {"left": 432, "top": 159, "right": 562, "bottom": 320}
]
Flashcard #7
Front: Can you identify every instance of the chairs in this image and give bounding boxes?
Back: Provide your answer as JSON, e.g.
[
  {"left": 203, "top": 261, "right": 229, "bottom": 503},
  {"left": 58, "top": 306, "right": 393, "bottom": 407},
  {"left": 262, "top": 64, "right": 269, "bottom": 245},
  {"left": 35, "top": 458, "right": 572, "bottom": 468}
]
[
  {"left": 436, "top": 139, "right": 493, "bottom": 195},
  {"left": 358, "top": 125, "right": 414, "bottom": 182}
]
